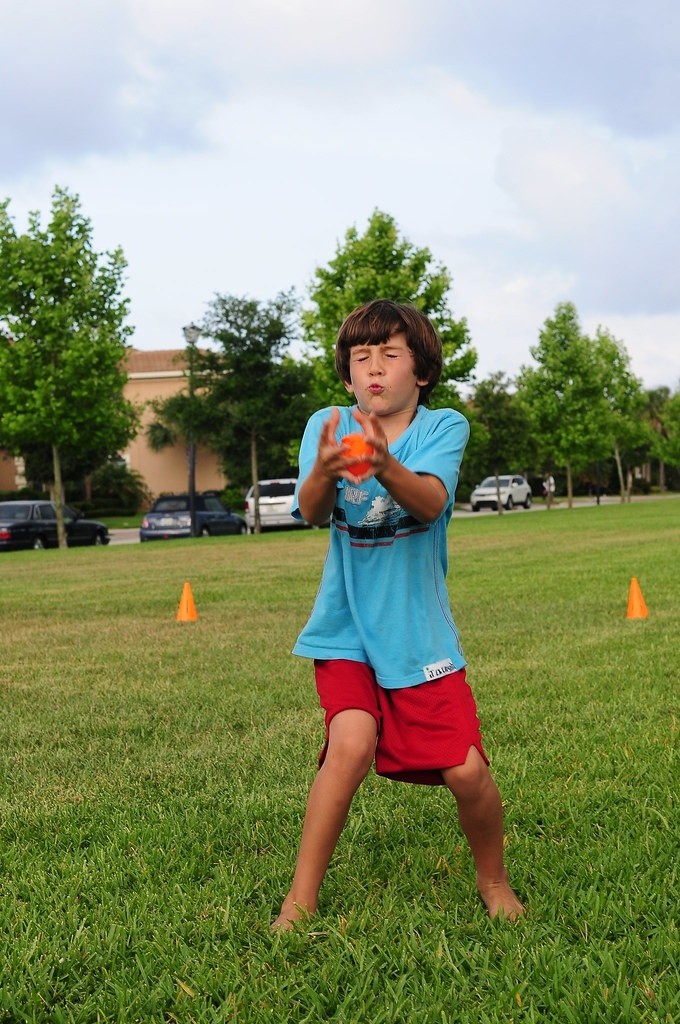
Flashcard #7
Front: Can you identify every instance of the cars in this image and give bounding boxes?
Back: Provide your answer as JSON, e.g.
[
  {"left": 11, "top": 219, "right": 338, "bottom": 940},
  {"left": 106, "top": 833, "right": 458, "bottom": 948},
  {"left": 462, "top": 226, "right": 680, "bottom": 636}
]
[
  {"left": 140, "top": 496, "right": 248, "bottom": 541},
  {"left": 0, "top": 501, "right": 111, "bottom": 552}
]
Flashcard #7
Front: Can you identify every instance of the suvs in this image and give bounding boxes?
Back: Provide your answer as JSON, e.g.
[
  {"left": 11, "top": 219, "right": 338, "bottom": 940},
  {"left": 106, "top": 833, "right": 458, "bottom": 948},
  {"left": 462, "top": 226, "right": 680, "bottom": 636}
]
[{"left": 470, "top": 475, "right": 532, "bottom": 511}]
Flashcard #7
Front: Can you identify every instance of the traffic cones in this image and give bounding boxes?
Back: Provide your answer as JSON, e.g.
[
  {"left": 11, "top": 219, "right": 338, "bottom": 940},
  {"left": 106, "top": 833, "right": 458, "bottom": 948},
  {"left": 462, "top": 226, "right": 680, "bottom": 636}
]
[
  {"left": 176, "top": 582, "right": 199, "bottom": 621},
  {"left": 626, "top": 578, "right": 649, "bottom": 618}
]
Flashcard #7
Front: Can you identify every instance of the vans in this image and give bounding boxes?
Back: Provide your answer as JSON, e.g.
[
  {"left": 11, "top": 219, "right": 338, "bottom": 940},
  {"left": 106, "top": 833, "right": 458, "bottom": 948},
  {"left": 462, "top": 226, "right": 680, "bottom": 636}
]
[{"left": 244, "top": 478, "right": 330, "bottom": 534}]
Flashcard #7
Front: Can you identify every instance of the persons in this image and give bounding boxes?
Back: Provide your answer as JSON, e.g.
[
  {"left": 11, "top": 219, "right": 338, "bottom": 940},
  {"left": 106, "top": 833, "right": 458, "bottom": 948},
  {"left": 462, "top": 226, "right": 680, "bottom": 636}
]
[
  {"left": 266, "top": 299, "right": 530, "bottom": 936},
  {"left": 543, "top": 472, "right": 561, "bottom": 508}
]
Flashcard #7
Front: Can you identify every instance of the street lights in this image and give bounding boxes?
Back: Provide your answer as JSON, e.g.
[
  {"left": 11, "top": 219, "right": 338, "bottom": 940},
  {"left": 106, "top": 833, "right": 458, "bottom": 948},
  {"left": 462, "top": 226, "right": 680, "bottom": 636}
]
[{"left": 182, "top": 322, "right": 203, "bottom": 538}]
